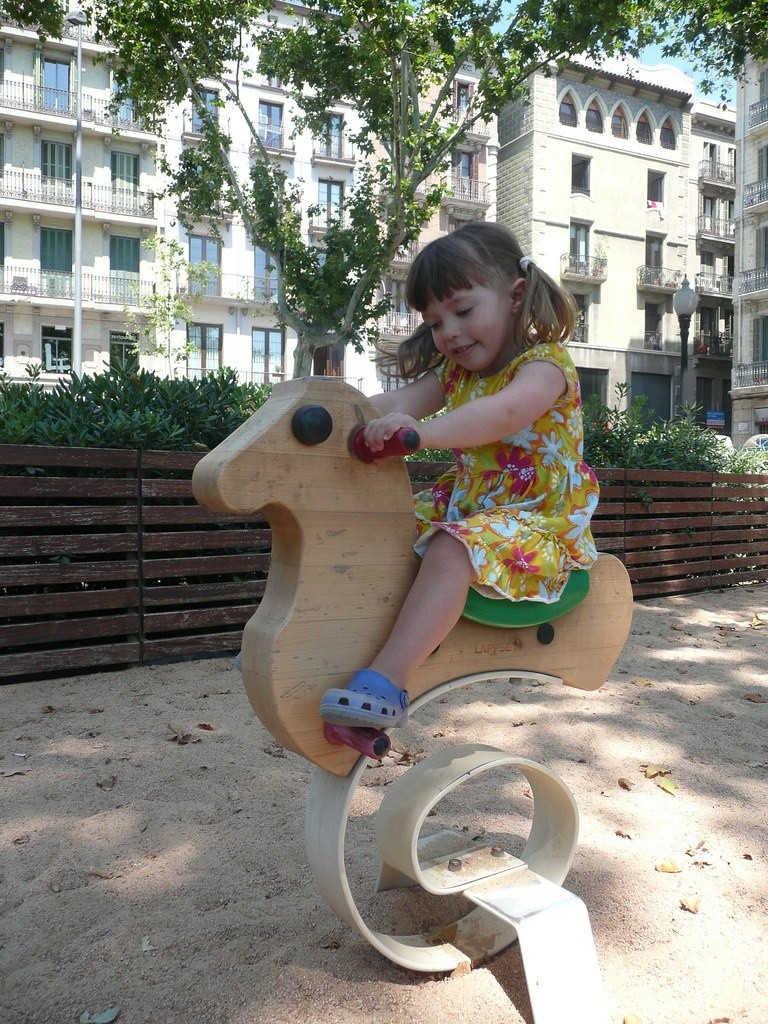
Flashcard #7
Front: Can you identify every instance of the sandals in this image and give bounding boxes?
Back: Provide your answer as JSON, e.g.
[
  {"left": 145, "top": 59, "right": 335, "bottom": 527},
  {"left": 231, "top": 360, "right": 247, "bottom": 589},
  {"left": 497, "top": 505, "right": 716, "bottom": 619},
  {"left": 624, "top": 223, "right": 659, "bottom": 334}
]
[
  {"left": 233, "top": 651, "right": 241, "bottom": 672},
  {"left": 320, "top": 668, "right": 409, "bottom": 727}
]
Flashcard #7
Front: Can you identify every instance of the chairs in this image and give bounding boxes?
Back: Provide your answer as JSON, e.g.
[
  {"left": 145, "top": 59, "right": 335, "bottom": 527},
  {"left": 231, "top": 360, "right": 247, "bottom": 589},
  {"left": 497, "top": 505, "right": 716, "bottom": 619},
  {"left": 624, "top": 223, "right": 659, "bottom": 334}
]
[{"left": 11, "top": 277, "right": 37, "bottom": 295}]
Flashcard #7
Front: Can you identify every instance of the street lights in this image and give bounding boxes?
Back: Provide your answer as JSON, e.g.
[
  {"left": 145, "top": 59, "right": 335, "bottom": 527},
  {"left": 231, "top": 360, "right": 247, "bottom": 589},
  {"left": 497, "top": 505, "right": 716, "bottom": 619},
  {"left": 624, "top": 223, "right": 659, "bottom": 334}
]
[
  {"left": 672, "top": 273, "right": 699, "bottom": 426},
  {"left": 66, "top": 9, "right": 88, "bottom": 400}
]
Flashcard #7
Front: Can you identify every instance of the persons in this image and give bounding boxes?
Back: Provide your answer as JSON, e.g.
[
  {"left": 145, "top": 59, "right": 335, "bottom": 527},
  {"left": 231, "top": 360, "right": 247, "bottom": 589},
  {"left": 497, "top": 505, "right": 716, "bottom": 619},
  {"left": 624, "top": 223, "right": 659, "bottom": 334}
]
[{"left": 317, "top": 221, "right": 603, "bottom": 729}]
